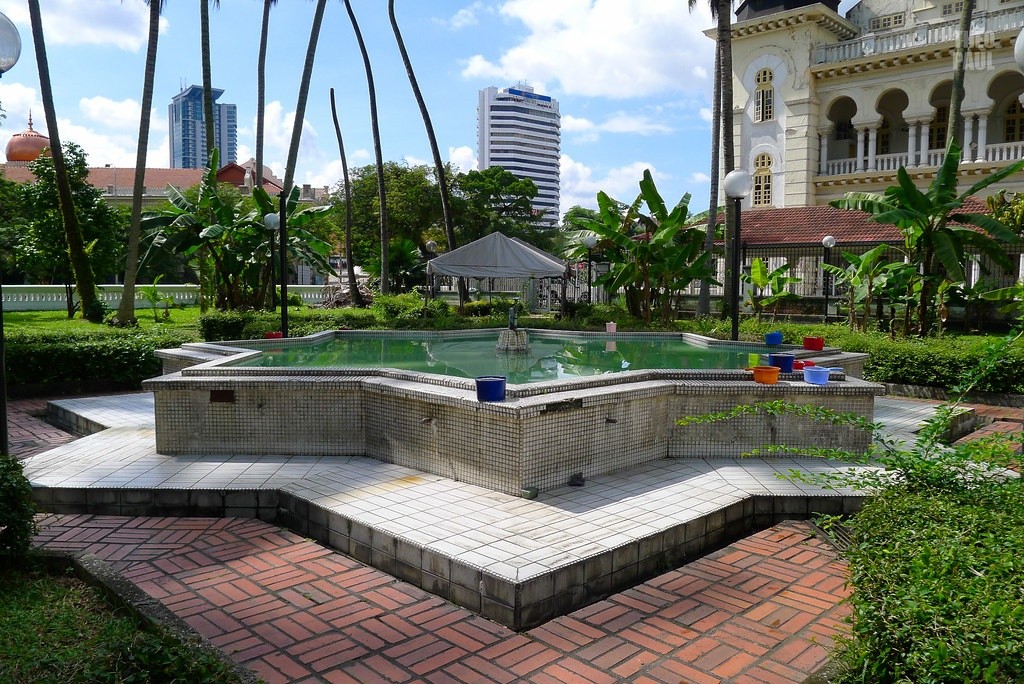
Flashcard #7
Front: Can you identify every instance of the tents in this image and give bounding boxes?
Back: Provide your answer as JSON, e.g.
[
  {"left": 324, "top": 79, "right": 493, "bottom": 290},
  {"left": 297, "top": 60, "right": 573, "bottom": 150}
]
[
  {"left": 421, "top": 230, "right": 567, "bottom": 330},
  {"left": 488, "top": 236, "right": 569, "bottom": 305}
]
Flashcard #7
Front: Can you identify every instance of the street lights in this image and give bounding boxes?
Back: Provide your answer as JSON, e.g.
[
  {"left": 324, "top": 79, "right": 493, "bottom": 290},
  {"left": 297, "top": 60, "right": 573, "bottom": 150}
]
[
  {"left": 821, "top": 235, "right": 835, "bottom": 324},
  {"left": 722, "top": 168, "right": 753, "bottom": 340},
  {"left": 583, "top": 236, "right": 596, "bottom": 305},
  {"left": 425, "top": 240, "right": 438, "bottom": 301},
  {"left": 264, "top": 213, "right": 281, "bottom": 312},
  {"left": 321, "top": 185, "right": 343, "bottom": 284},
  {"left": 105, "top": 163, "right": 119, "bottom": 285}
]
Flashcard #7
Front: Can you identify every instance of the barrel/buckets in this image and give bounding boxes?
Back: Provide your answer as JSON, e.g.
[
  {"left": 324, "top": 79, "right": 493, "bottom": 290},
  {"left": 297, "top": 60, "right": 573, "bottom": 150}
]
[
  {"left": 768, "top": 352, "right": 794, "bottom": 373},
  {"left": 803, "top": 335, "right": 824, "bottom": 351},
  {"left": 803, "top": 365, "right": 830, "bottom": 385},
  {"left": 765, "top": 332, "right": 783, "bottom": 345},
  {"left": 475, "top": 374, "right": 508, "bottom": 402},
  {"left": 754, "top": 364, "right": 781, "bottom": 385}
]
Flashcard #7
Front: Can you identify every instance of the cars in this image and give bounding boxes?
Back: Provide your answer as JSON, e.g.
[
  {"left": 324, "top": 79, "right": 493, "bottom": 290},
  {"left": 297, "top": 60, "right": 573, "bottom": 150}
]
[{"left": 329, "top": 255, "right": 348, "bottom": 269}]
